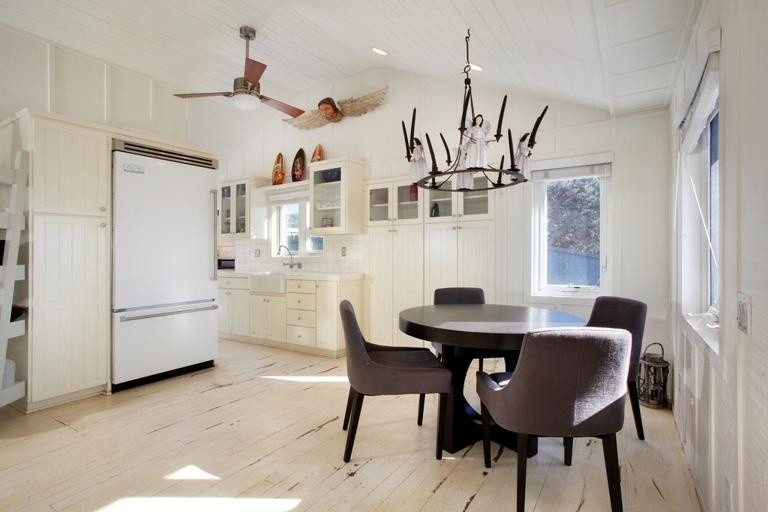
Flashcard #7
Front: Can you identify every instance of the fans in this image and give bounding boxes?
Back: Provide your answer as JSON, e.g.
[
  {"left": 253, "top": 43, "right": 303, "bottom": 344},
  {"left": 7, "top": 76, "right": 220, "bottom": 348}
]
[{"left": 173, "top": 26, "right": 305, "bottom": 118}]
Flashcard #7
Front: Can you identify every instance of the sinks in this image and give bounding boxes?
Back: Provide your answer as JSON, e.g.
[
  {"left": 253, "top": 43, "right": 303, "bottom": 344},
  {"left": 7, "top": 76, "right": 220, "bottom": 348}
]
[{"left": 250, "top": 271, "right": 306, "bottom": 295}]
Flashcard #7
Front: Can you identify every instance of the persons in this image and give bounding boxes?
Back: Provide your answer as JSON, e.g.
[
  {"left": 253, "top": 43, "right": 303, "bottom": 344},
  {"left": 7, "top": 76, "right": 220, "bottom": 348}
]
[
  {"left": 318, "top": 98, "right": 339, "bottom": 118},
  {"left": 463, "top": 114, "right": 495, "bottom": 169},
  {"left": 408, "top": 137, "right": 428, "bottom": 182},
  {"left": 514, "top": 132, "right": 532, "bottom": 181}
]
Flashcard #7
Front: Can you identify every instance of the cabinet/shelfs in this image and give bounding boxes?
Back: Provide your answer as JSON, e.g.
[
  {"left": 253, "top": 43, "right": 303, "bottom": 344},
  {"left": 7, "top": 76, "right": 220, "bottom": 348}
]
[
  {"left": 362, "top": 177, "right": 424, "bottom": 347},
  {"left": 218, "top": 273, "right": 249, "bottom": 342},
  {"left": 249, "top": 294, "right": 286, "bottom": 349},
  {"left": 217, "top": 176, "right": 269, "bottom": 240},
  {"left": 308, "top": 157, "right": 368, "bottom": 236},
  {"left": 424, "top": 172, "right": 496, "bottom": 368},
  {"left": 287, "top": 274, "right": 363, "bottom": 354},
  {"left": 0, "top": 108, "right": 112, "bottom": 414}
]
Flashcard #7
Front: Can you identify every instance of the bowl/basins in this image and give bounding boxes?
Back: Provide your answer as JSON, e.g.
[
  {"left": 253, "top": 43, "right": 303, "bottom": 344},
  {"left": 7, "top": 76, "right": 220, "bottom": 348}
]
[{"left": 323, "top": 171, "right": 340, "bottom": 182}]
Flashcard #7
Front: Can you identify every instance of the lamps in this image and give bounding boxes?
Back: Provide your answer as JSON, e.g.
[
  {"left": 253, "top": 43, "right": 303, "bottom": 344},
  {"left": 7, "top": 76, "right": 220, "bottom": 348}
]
[
  {"left": 401, "top": 30, "right": 548, "bottom": 193},
  {"left": 232, "top": 26, "right": 261, "bottom": 110}
]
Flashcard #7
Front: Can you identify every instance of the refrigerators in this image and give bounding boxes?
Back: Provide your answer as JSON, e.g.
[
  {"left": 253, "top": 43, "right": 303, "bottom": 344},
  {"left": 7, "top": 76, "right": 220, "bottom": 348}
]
[{"left": 110, "top": 150, "right": 220, "bottom": 388}]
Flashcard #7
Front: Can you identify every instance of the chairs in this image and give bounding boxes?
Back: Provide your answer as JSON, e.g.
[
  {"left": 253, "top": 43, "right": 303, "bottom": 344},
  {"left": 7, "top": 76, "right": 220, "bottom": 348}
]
[
  {"left": 476, "top": 328, "right": 633, "bottom": 511},
  {"left": 339, "top": 300, "right": 451, "bottom": 462},
  {"left": 563, "top": 297, "right": 644, "bottom": 464},
  {"left": 418, "top": 288, "right": 485, "bottom": 425}
]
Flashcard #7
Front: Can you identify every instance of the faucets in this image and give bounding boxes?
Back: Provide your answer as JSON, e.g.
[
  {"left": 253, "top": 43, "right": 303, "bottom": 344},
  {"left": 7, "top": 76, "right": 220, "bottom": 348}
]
[{"left": 277, "top": 245, "right": 293, "bottom": 265}]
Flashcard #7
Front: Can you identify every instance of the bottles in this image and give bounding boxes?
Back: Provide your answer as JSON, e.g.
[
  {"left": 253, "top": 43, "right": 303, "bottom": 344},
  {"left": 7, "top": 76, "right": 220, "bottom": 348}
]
[
  {"left": 409, "top": 185, "right": 417, "bottom": 201},
  {"left": 429, "top": 203, "right": 439, "bottom": 217}
]
